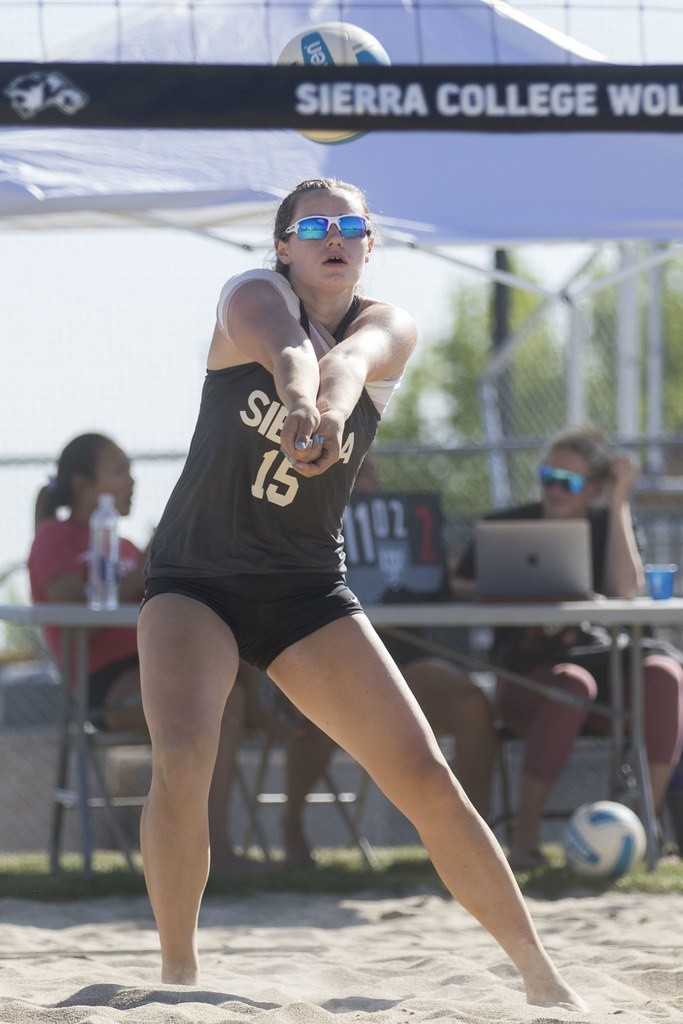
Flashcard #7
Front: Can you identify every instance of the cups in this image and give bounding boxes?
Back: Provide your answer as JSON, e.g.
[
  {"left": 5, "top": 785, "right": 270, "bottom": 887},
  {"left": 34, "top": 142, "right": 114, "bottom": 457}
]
[{"left": 646, "top": 564, "right": 679, "bottom": 599}]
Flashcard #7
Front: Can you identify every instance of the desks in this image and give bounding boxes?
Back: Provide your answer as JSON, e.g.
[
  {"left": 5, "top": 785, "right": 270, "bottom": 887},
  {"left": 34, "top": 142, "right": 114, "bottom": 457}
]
[{"left": 0, "top": 598, "right": 683, "bottom": 885}]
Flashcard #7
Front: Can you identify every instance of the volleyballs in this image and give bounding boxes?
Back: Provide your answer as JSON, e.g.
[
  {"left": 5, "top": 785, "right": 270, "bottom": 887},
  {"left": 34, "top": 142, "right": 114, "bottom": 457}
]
[
  {"left": 278, "top": 21, "right": 393, "bottom": 144},
  {"left": 561, "top": 799, "right": 646, "bottom": 879}
]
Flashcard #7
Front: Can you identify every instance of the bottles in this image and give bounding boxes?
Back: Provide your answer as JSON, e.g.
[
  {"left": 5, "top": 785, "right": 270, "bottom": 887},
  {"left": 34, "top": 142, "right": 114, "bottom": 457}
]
[{"left": 86, "top": 494, "right": 120, "bottom": 612}]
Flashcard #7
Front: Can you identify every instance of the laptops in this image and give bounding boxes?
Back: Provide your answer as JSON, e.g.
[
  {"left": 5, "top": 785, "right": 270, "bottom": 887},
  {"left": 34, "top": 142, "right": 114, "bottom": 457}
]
[{"left": 473, "top": 522, "right": 593, "bottom": 603}]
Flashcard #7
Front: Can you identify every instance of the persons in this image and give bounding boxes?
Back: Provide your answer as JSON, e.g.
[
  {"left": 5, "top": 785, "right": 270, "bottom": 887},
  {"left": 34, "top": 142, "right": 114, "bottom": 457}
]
[
  {"left": 134, "top": 176, "right": 590, "bottom": 1012},
  {"left": 452, "top": 433, "right": 683, "bottom": 877},
  {"left": 27, "top": 431, "right": 280, "bottom": 886},
  {"left": 280, "top": 448, "right": 500, "bottom": 891}
]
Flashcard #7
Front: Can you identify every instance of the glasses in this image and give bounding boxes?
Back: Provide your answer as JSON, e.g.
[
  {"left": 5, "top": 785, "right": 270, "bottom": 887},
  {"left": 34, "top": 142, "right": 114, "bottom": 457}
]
[
  {"left": 279, "top": 214, "right": 371, "bottom": 240},
  {"left": 537, "top": 462, "right": 603, "bottom": 492}
]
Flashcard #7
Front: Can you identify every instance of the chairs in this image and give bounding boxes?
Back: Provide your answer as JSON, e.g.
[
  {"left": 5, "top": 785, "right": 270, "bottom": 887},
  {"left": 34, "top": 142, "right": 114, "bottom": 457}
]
[{"left": 0, "top": 562, "right": 276, "bottom": 887}]
